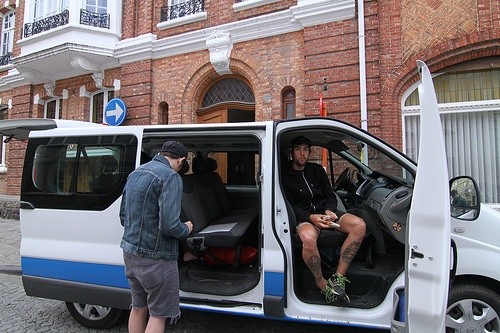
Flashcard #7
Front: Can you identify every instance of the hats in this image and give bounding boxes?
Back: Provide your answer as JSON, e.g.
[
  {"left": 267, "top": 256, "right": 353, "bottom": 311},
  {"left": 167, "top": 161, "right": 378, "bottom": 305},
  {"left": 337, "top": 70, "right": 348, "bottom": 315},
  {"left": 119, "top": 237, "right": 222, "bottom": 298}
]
[
  {"left": 160, "top": 141, "right": 190, "bottom": 174},
  {"left": 292, "top": 135, "right": 313, "bottom": 146}
]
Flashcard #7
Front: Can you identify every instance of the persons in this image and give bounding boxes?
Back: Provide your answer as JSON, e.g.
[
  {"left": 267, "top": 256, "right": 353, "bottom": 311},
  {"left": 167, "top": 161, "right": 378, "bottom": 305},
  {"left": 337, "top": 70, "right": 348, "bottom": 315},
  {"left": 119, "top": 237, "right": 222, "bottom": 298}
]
[
  {"left": 119, "top": 140, "right": 193, "bottom": 332},
  {"left": 451, "top": 189, "right": 468, "bottom": 206},
  {"left": 283, "top": 136, "right": 366, "bottom": 305}
]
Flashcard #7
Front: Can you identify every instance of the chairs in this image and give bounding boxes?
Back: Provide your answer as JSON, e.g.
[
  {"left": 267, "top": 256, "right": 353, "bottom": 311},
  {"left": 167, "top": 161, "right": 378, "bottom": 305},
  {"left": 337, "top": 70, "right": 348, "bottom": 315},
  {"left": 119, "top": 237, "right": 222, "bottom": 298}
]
[
  {"left": 283, "top": 178, "right": 373, "bottom": 272},
  {"left": 90, "top": 154, "right": 260, "bottom": 272}
]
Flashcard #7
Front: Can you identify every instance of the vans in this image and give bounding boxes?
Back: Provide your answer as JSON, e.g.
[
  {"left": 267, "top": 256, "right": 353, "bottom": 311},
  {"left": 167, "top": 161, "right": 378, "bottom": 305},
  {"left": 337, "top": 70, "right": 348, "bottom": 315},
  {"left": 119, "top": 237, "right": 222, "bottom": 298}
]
[{"left": 0, "top": 58, "right": 500, "bottom": 333}]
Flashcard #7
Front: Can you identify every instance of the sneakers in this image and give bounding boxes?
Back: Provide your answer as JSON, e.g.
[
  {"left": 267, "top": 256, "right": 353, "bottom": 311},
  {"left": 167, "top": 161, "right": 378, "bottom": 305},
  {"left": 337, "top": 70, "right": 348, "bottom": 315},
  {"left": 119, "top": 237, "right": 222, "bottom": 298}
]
[
  {"left": 320, "top": 285, "right": 350, "bottom": 308},
  {"left": 328, "top": 272, "right": 351, "bottom": 301}
]
